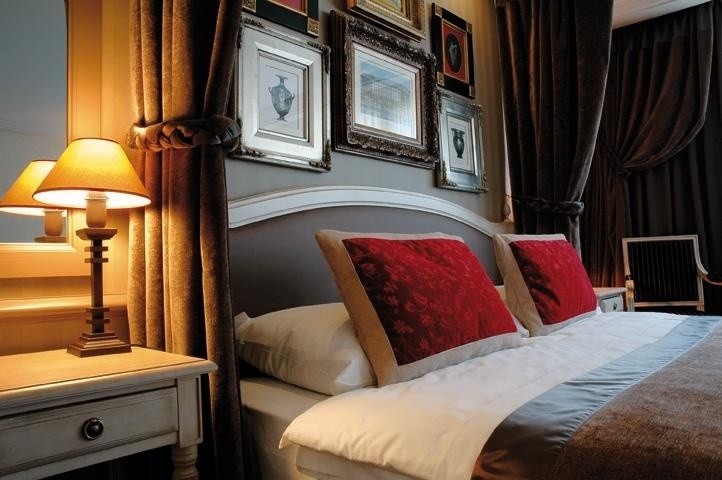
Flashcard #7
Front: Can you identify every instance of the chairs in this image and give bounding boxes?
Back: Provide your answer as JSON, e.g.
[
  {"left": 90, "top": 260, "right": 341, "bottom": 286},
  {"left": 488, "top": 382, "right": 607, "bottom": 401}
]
[{"left": 619, "top": 233, "right": 713, "bottom": 317}]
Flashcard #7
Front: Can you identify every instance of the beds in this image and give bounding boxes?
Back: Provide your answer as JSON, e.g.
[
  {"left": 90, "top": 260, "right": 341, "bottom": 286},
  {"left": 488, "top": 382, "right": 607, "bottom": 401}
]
[{"left": 202, "top": 181, "right": 722, "bottom": 480}]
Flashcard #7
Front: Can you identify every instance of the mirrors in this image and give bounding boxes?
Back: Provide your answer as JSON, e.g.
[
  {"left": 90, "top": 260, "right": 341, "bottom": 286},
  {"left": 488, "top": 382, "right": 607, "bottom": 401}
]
[{"left": 0, "top": 0, "right": 103, "bottom": 281}]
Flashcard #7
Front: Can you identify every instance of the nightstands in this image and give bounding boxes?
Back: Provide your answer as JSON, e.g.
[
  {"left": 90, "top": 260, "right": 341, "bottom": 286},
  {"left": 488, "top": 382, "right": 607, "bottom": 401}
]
[
  {"left": 0, "top": 343, "right": 219, "bottom": 479},
  {"left": 591, "top": 286, "right": 628, "bottom": 315}
]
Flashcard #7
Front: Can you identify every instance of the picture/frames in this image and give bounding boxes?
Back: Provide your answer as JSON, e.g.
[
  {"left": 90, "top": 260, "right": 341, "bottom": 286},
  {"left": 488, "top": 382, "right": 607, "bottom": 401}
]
[
  {"left": 228, "top": 11, "right": 334, "bottom": 176},
  {"left": 428, "top": 1, "right": 479, "bottom": 99},
  {"left": 344, "top": 0, "right": 430, "bottom": 41},
  {"left": 327, "top": 8, "right": 442, "bottom": 173},
  {"left": 240, "top": 0, "right": 322, "bottom": 38},
  {"left": 432, "top": 85, "right": 491, "bottom": 197}
]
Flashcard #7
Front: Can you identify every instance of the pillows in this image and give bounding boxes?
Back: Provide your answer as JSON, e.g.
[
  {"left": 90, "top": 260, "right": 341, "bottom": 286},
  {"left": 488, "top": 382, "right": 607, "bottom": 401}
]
[
  {"left": 490, "top": 231, "right": 600, "bottom": 339},
  {"left": 229, "top": 299, "right": 376, "bottom": 401},
  {"left": 493, "top": 279, "right": 535, "bottom": 340},
  {"left": 311, "top": 226, "right": 527, "bottom": 389}
]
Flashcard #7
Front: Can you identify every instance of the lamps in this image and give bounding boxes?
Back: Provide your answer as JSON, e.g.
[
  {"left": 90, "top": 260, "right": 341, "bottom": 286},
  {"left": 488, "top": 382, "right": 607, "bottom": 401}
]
[
  {"left": 32, "top": 135, "right": 153, "bottom": 358},
  {"left": 0, "top": 158, "right": 67, "bottom": 249}
]
